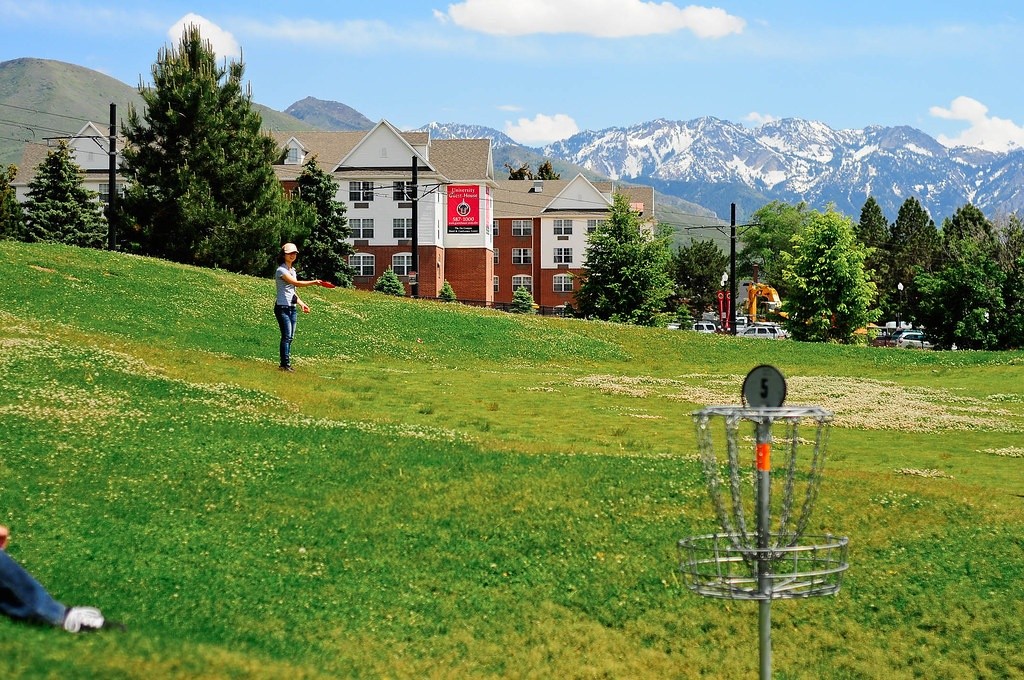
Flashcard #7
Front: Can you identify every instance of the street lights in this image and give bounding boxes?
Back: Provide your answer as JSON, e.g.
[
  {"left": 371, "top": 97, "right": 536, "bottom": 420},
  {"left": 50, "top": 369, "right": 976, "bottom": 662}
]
[{"left": 898, "top": 282, "right": 903, "bottom": 328}]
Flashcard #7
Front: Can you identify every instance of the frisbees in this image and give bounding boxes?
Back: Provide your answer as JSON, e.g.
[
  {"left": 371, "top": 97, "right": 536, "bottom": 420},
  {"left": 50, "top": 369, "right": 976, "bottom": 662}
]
[{"left": 319, "top": 281, "right": 336, "bottom": 289}]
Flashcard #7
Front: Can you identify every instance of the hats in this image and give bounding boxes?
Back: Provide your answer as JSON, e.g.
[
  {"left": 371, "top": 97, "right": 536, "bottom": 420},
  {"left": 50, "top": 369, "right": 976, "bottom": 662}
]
[{"left": 282, "top": 243, "right": 299, "bottom": 254}]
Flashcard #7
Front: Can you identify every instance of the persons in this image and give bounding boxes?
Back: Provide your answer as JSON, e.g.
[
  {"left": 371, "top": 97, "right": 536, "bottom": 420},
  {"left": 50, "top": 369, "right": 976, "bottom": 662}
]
[
  {"left": 0, "top": 524, "right": 105, "bottom": 633},
  {"left": 274, "top": 242, "right": 322, "bottom": 372}
]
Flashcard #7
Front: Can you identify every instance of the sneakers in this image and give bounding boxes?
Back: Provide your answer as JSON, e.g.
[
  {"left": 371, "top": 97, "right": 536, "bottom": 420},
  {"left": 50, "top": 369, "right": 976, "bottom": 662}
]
[{"left": 63, "top": 606, "right": 125, "bottom": 635}]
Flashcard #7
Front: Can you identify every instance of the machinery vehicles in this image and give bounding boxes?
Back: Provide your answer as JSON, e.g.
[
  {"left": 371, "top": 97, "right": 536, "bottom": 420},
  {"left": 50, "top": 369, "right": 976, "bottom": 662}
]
[{"left": 748, "top": 283, "right": 789, "bottom": 323}]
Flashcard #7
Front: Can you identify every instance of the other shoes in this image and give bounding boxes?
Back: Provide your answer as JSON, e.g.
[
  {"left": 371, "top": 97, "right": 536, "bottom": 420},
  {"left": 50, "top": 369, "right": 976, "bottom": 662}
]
[{"left": 279, "top": 365, "right": 295, "bottom": 372}]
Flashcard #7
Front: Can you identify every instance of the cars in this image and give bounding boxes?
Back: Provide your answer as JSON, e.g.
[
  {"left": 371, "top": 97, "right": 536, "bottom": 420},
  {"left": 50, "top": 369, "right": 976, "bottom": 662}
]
[
  {"left": 693, "top": 323, "right": 716, "bottom": 333},
  {"left": 736, "top": 325, "right": 785, "bottom": 338},
  {"left": 898, "top": 333, "right": 935, "bottom": 349},
  {"left": 867, "top": 326, "right": 923, "bottom": 347},
  {"left": 718, "top": 317, "right": 748, "bottom": 332}
]
